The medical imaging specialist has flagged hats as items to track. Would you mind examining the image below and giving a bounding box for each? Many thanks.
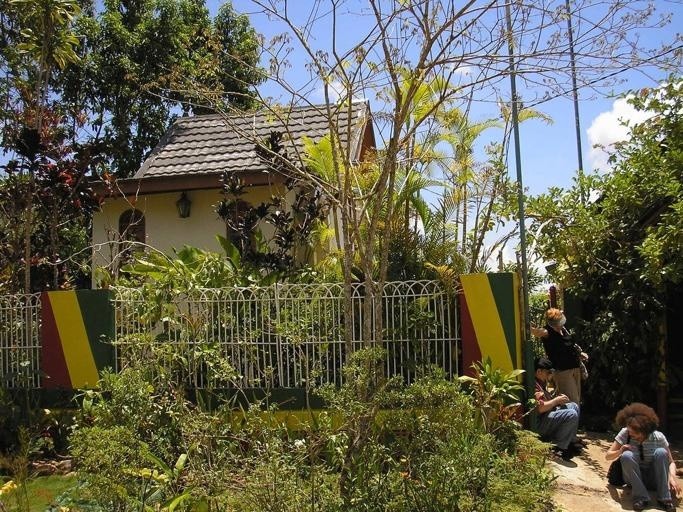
[534,358,556,371]
[548,312,566,326]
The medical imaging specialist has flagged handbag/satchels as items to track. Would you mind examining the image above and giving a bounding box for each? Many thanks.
[606,455,626,486]
[579,360,588,380]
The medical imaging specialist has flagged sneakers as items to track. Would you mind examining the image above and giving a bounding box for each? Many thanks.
[657,500,676,512]
[632,501,649,511]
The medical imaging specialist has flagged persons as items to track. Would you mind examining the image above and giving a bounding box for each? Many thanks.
[604,403,683,512]
[534,357,581,461]
[531,306,589,434]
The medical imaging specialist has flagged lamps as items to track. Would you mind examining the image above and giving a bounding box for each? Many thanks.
[175,192,191,219]
